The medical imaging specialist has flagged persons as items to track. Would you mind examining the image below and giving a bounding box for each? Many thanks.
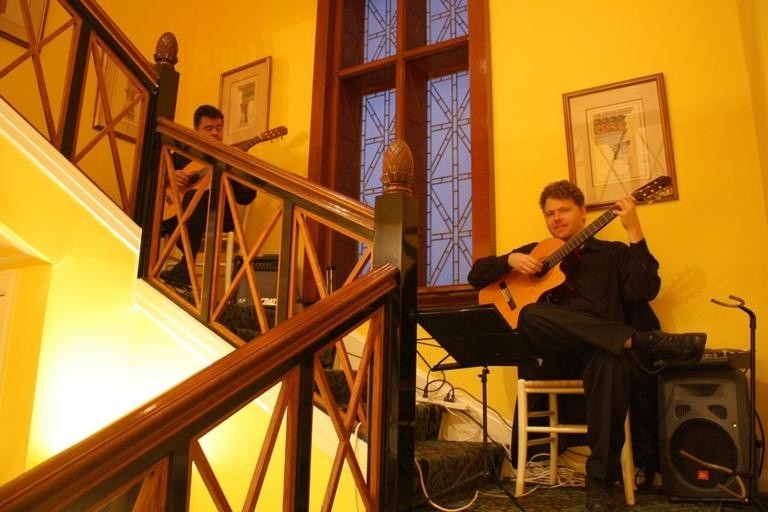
[467,180,707,512]
[160,105,256,292]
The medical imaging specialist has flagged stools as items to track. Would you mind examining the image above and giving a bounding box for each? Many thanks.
[515,379,636,505]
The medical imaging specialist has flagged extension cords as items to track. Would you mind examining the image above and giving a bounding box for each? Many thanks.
[415,396,469,411]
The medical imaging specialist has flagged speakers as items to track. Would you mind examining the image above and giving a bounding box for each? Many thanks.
[656,369,759,504]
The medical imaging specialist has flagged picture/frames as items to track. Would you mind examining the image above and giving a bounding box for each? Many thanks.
[0,0,50,52]
[218,54,272,146]
[92,51,142,143]
[562,71,681,211]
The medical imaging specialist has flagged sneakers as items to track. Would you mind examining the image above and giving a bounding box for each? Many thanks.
[586,489,619,512]
[635,329,706,366]
[159,258,190,284]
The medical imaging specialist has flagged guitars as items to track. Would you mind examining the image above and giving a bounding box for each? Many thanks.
[480,176,672,331]
[163,126,287,222]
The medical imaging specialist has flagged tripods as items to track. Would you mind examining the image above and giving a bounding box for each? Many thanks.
[412,369,526,512]
